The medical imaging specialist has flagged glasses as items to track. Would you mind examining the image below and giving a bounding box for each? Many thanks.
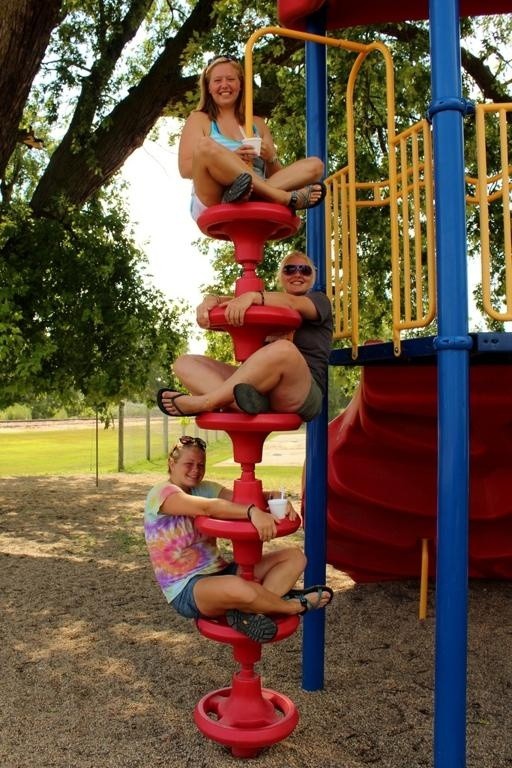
[282,264,313,276]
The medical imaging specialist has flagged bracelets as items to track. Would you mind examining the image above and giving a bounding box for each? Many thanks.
[269,492,272,499]
[247,504,255,521]
[259,291,264,306]
[212,295,221,304]
[268,154,278,164]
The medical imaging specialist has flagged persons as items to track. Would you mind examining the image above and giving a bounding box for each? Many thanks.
[158,252,333,422]
[178,57,326,223]
[144,436,334,644]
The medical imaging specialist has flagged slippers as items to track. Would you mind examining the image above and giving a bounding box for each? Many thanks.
[281,585,333,615]
[224,608,276,644]
[221,173,253,202]
[232,382,271,414]
[157,388,202,417]
[289,182,326,211]
